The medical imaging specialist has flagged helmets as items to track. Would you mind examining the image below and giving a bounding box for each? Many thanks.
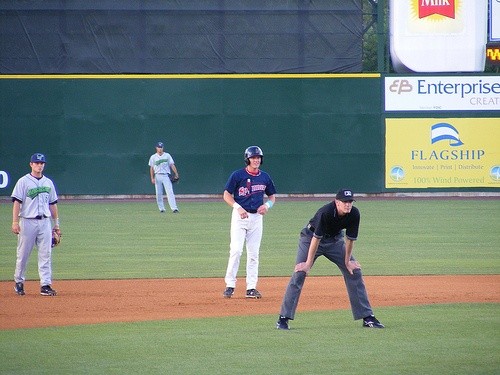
[244,146,264,165]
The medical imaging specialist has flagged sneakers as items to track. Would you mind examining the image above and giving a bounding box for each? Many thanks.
[276,316,290,330]
[223,287,234,297]
[363,315,384,328]
[14,282,25,296]
[40,285,57,295]
[246,289,261,297]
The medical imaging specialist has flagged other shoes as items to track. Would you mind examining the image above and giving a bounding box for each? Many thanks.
[161,210,165,213]
[174,210,179,213]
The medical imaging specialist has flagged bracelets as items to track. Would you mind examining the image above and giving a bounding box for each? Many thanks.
[12,222,19,225]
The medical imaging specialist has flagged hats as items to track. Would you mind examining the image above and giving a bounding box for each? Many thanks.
[31,153,46,163]
[336,189,355,201]
[156,142,164,147]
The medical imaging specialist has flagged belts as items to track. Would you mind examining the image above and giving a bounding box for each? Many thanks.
[307,223,315,232]
[36,216,46,219]
[247,210,257,213]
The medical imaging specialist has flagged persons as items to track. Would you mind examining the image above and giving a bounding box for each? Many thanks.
[277,188,385,331]
[222,146,277,299]
[9,153,62,296]
[148,142,180,213]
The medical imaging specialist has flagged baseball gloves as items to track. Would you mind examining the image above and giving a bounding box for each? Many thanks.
[52,229,63,248]
[169,171,182,184]
